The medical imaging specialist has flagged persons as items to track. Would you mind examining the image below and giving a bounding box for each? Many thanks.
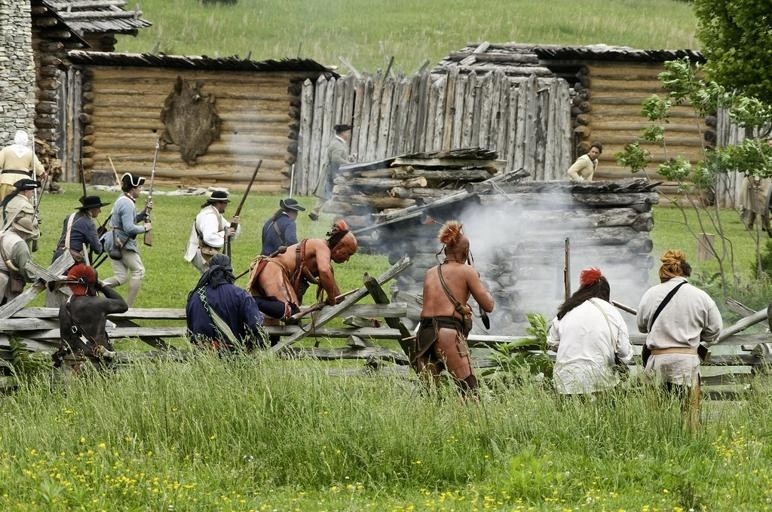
[308,125,354,221]
[546,267,634,405]
[261,198,305,257]
[44,196,111,308]
[186,253,268,361]
[566,143,602,180]
[59,263,128,376]
[637,250,723,428]
[100,172,153,308]
[0,179,41,256]
[0,130,46,202]
[0,216,38,307]
[415,218,495,404]
[184,191,241,275]
[246,220,358,348]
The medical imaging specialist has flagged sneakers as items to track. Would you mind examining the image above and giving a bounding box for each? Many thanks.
[309,213,318,220]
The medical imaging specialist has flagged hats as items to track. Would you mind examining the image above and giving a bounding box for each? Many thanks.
[121,172,146,186]
[210,191,230,203]
[12,217,34,235]
[74,196,110,209]
[14,180,41,191]
[334,125,353,132]
[280,199,307,213]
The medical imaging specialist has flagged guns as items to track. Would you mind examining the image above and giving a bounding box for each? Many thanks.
[610,300,711,362]
[460,227,489,329]
[89,214,112,264]
[24,136,39,216]
[144,141,160,246]
[285,288,359,324]
[223,160,262,265]
